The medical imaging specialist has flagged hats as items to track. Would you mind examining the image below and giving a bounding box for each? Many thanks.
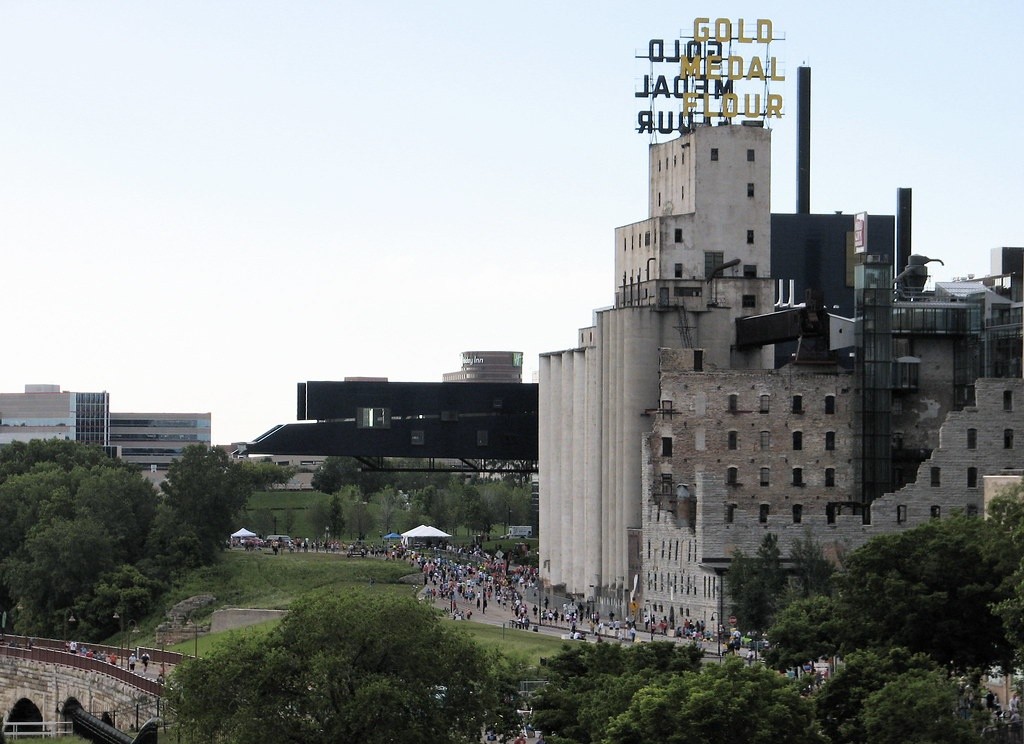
[132,653,134,656]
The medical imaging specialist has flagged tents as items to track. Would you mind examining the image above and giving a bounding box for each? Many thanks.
[384,533,401,542]
[402,525,452,540]
[231,527,257,548]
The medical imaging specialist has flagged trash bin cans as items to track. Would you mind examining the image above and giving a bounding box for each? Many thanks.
[532,625,538,632]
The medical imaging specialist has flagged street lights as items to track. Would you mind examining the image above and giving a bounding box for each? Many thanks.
[643,603,653,641]
[586,596,596,626]
[64,609,76,652]
[711,611,722,657]
[126,619,140,671]
[113,606,124,667]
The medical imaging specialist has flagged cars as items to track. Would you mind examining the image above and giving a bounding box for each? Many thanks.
[267,534,292,547]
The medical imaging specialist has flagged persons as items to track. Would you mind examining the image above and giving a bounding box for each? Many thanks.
[746,648,753,667]
[472,530,510,541]
[585,605,604,632]
[226,537,357,558]
[561,601,584,629]
[625,614,636,642]
[544,596,549,609]
[65,640,150,674]
[608,610,615,630]
[362,541,539,620]
[614,620,622,631]
[957,685,1024,744]
[644,615,648,630]
[723,629,741,657]
[762,635,770,649]
[651,614,656,636]
[676,618,712,645]
[568,629,587,641]
[658,616,668,637]
[785,658,829,693]
[533,605,559,626]
[494,582,530,631]
